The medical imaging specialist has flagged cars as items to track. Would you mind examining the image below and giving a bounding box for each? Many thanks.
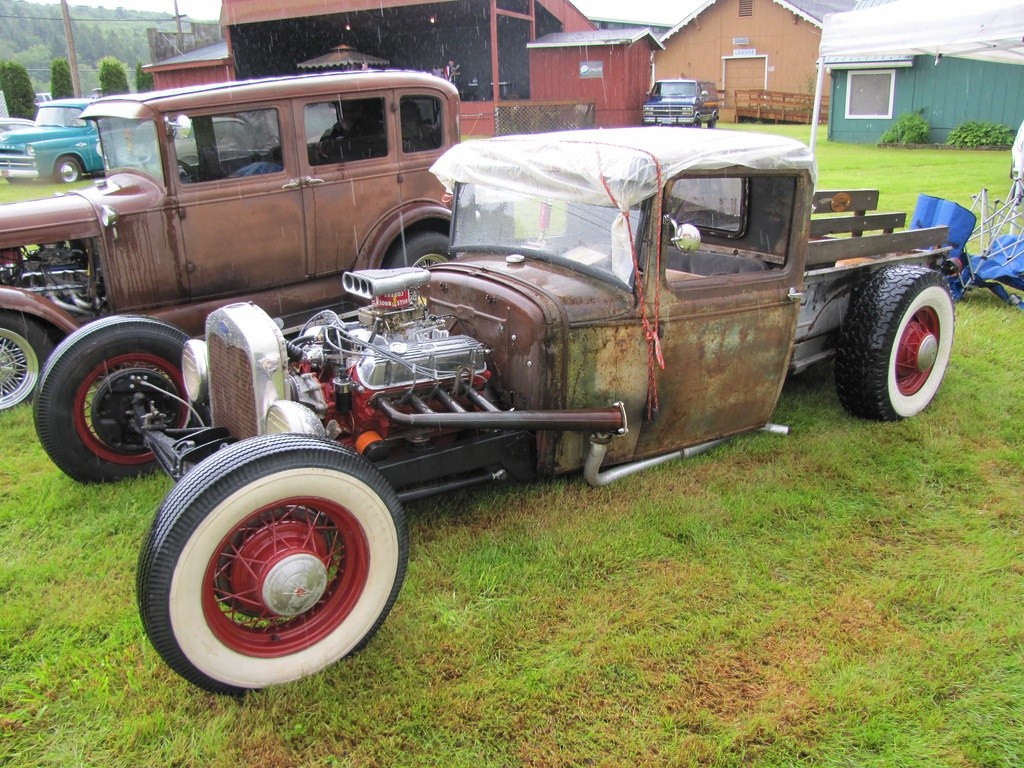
[31,91,55,105]
[0,97,172,185]
[0,66,470,413]
[0,117,35,146]
[32,128,954,693]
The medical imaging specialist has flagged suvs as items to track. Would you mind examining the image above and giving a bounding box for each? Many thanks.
[638,79,722,134]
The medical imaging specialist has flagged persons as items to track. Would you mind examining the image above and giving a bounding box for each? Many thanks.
[226,143,285,180]
